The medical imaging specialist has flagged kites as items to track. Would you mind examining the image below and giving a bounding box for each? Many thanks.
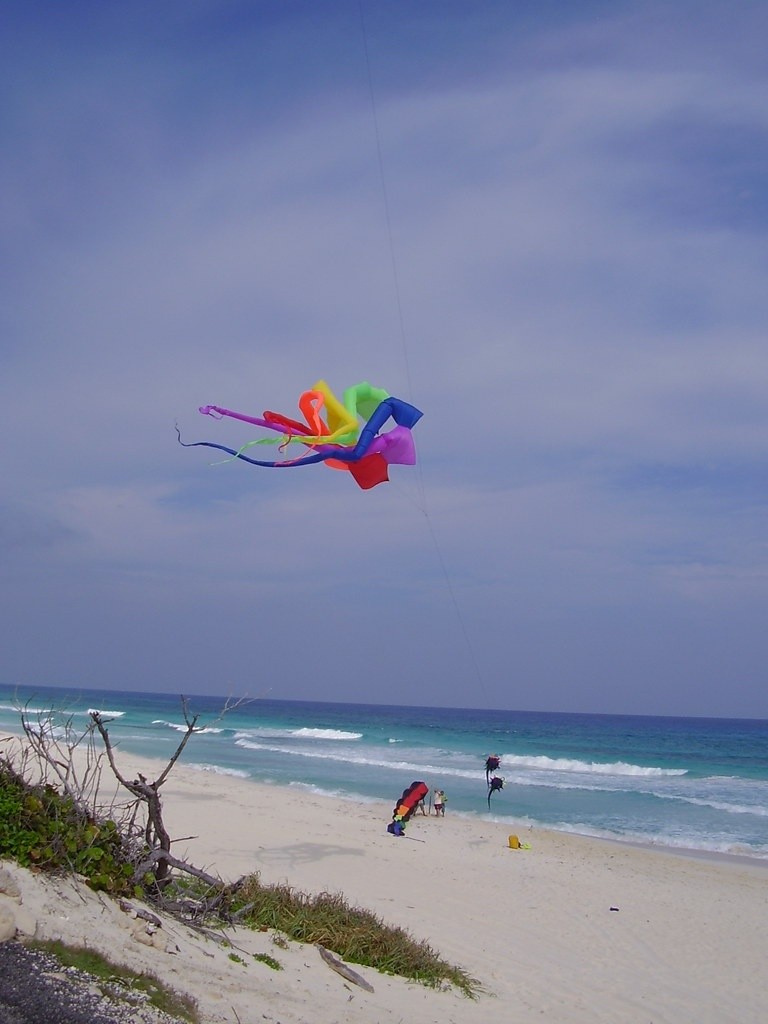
[175,380,424,490]
[487,775,504,810]
[483,756,500,788]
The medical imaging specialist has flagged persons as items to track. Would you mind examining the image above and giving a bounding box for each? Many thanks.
[392,819,405,836]
[413,798,427,816]
[434,788,447,817]
